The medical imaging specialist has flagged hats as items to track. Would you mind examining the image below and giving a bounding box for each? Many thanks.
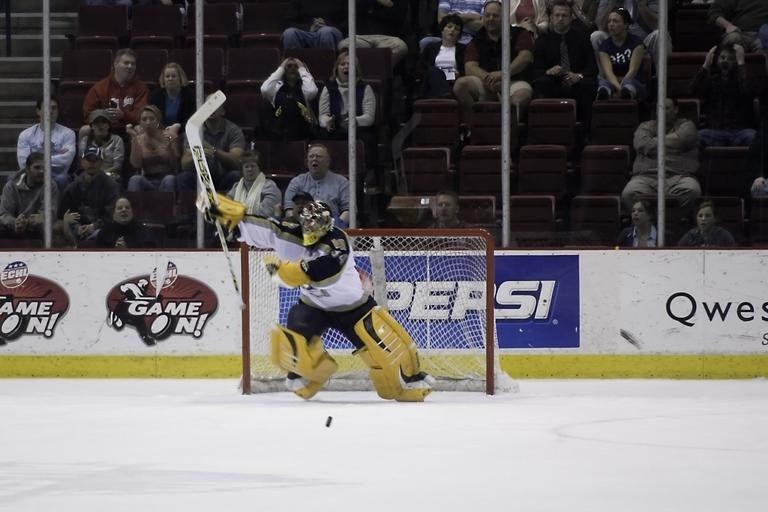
[292,191,313,203]
[89,110,110,125]
[82,146,103,161]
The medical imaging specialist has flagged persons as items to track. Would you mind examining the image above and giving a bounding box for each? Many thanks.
[1,0,767,262]
[205,200,436,391]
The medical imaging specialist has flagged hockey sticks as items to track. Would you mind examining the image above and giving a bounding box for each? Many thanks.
[186,90,246,311]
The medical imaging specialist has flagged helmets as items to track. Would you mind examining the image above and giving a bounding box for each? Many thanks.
[301,199,335,246]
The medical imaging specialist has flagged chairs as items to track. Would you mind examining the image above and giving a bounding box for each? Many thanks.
[54,0,767,247]
